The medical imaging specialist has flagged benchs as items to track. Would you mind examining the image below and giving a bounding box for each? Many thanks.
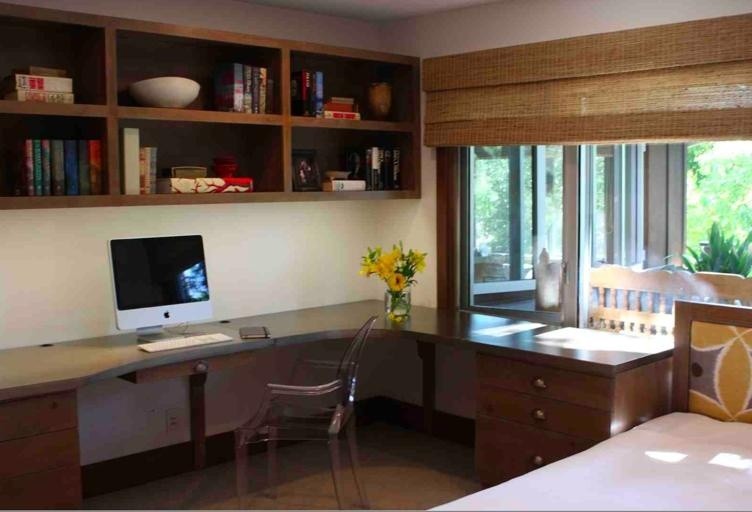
[534,249,752,340]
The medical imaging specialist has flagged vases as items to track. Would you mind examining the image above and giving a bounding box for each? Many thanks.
[384,289,412,323]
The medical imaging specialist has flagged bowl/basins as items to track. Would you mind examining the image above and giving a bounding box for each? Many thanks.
[130,75,200,108]
[214,150,241,178]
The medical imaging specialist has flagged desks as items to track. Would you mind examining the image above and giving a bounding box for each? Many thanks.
[0,298,676,511]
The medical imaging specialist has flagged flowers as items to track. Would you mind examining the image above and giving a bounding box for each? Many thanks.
[361,240,428,313]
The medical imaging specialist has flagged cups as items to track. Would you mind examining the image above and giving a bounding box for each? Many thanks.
[369,83,394,123]
[535,261,562,312]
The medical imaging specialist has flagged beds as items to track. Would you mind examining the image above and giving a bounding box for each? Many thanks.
[428,298,751,512]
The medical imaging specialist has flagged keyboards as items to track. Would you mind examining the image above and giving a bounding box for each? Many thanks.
[137,333,233,352]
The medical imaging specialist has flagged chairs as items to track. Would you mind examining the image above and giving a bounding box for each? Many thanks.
[233,314,378,512]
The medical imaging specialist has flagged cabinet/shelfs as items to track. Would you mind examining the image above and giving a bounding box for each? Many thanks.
[0,2,421,212]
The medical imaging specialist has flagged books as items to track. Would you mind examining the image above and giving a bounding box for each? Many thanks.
[4,91,76,104]
[2,127,254,197]
[239,326,271,339]
[322,146,400,191]
[12,65,76,78]
[1,75,75,92]
[214,62,361,120]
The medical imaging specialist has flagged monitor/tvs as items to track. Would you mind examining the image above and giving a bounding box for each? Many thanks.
[108,234,210,341]
